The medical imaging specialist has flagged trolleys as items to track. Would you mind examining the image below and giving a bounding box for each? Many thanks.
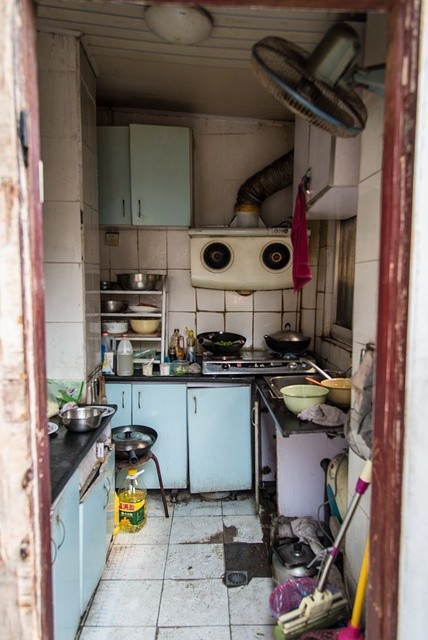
[249,23,388,137]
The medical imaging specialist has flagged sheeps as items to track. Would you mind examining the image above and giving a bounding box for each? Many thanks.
[299,358,332,379]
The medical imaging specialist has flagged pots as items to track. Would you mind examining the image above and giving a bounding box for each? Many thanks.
[197,331,246,355]
[264,323,311,353]
[111,424,159,465]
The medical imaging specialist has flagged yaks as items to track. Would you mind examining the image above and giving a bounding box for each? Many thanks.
[299,520,371,640]
[273,459,372,640]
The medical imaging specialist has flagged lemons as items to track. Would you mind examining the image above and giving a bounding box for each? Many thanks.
[271,542,319,587]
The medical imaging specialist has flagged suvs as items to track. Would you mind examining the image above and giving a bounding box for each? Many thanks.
[101,331,116,376]
[117,468,147,533]
[170,329,184,360]
[116,333,134,377]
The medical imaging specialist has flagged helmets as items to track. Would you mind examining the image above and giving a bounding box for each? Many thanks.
[128,305,158,313]
[83,406,115,417]
[47,422,58,435]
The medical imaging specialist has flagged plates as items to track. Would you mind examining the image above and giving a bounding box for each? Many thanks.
[186,386,253,495]
[95,124,192,228]
[79,440,116,615]
[292,114,361,221]
[98,284,167,364]
[50,467,81,640]
[100,383,187,491]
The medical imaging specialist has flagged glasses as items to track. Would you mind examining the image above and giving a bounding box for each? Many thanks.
[143,4,213,45]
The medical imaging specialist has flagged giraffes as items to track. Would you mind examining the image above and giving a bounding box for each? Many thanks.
[202,350,317,375]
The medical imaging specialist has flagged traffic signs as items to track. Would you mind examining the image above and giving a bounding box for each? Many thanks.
[105,233,119,246]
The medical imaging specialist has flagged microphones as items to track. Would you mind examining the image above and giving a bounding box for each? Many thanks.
[116,450,169,518]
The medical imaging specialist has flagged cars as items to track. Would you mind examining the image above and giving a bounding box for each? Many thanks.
[161,363,170,375]
[143,360,152,376]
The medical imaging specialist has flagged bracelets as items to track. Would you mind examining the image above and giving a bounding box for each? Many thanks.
[99,280,116,290]
[116,273,166,290]
[101,321,128,334]
[280,385,329,415]
[130,320,160,334]
[100,300,129,313]
[59,407,105,432]
[321,378,351,404]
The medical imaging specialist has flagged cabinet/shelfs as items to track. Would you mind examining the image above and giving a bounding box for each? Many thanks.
[119,468,148,533]
[186,328,197,362]
[117,334,134,376]
[170,329,184,361]
[101,331,116,375]
[113,491,118,535]
